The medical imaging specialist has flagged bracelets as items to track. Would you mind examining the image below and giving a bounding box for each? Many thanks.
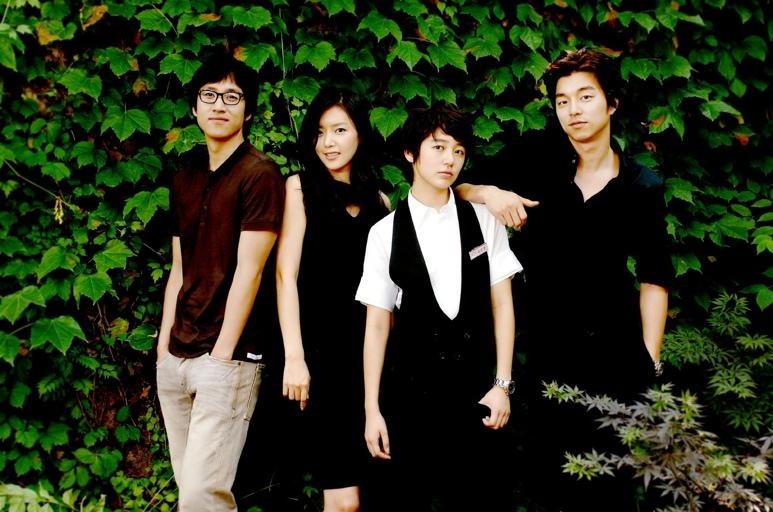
[653,357,665,377]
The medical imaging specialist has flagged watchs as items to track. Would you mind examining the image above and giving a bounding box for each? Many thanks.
[491,377,517,395]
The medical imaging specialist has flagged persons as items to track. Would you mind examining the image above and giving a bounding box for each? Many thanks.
[452,48,677,512]
[152,55,288,511]
[273,82,393,512]
[353,105,523,512]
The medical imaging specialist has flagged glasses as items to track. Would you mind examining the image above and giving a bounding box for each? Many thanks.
[194,89,246,105]
